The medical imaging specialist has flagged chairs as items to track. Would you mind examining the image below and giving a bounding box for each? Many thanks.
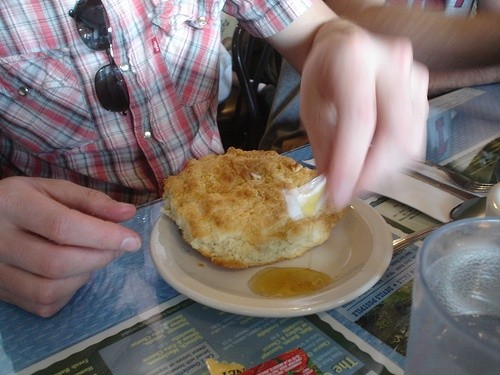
[232,22,282,151]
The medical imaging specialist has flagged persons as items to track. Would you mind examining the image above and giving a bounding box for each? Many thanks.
[259,1,500,156]
[0,0,431,318]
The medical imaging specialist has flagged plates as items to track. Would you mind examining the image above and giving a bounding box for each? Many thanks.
[150,198,393,317]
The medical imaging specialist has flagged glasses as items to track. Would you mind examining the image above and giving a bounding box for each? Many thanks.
[68,0,130,116]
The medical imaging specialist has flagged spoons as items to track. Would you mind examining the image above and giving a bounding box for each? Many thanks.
[393,197,486,254]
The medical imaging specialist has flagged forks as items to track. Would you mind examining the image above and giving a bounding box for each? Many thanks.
[415,159,493,190]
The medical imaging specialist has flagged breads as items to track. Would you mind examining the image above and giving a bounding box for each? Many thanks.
[160,147,353,267]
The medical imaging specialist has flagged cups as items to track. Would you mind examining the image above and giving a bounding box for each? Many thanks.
[405,216,500,375]
